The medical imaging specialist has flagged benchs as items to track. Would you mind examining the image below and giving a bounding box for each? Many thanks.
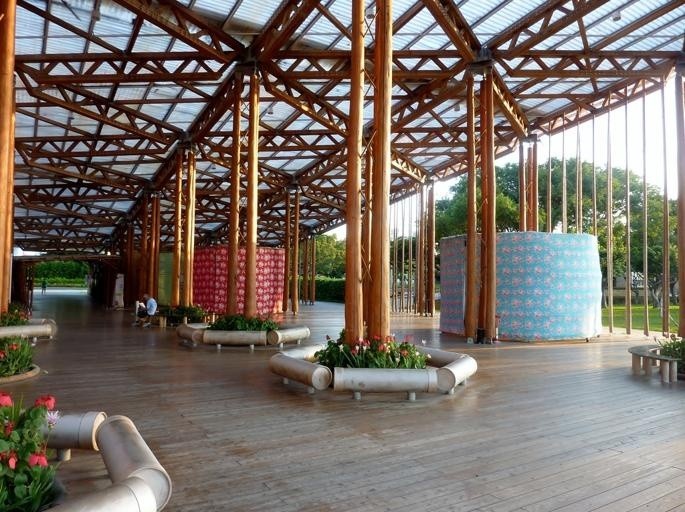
[627,344,683,383]
[153,312,168,328]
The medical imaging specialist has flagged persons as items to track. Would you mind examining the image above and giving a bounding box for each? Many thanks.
[41,278,48,296]
[131,291,158,328]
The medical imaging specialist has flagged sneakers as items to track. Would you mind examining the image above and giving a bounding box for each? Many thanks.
[144,323,151,327]
[132,322,138,326]
